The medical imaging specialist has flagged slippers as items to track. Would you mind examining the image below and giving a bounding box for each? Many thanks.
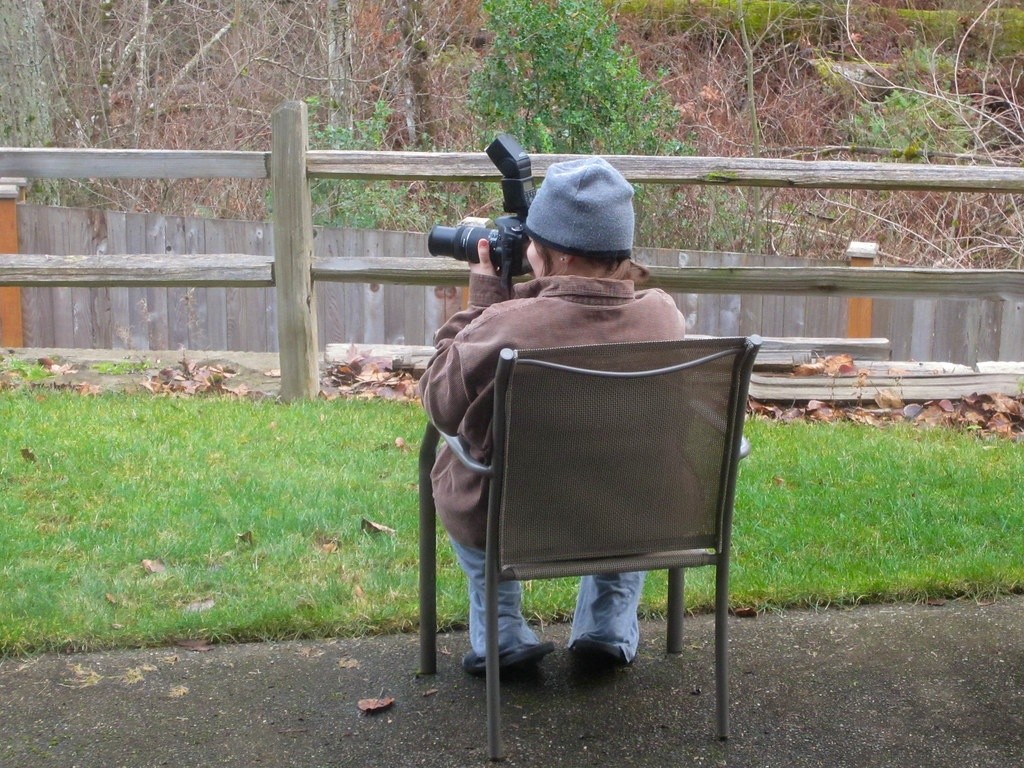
[572,639,626,665]
[461,640,554,673]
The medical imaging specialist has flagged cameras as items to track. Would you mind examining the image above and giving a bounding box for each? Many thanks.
[428,135,539,277]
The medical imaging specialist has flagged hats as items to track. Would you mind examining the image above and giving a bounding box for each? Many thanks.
[526,158,634,257]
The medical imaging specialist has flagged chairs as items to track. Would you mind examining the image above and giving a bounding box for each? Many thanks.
[418,333,765,761]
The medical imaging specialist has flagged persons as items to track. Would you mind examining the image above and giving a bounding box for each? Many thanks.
[416,156,686,674]
